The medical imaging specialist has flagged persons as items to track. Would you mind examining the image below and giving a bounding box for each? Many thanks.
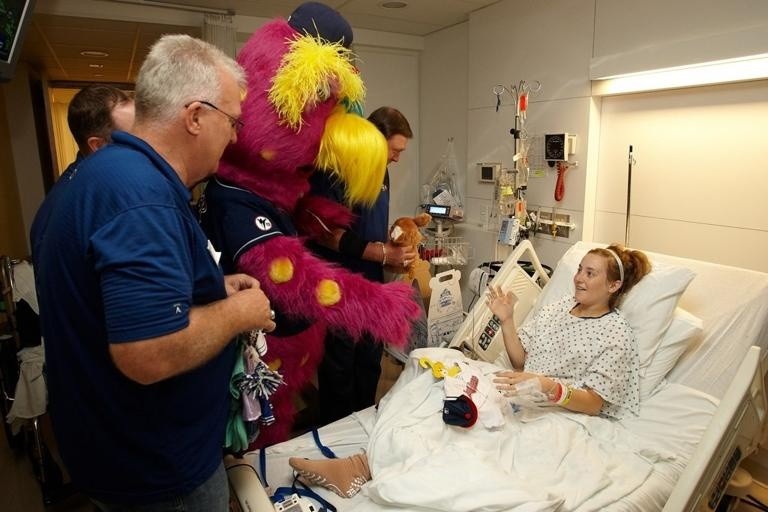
[27,32,279,512]
[312,107,416,423]
[289,243,651,498]
[51,84,136,186]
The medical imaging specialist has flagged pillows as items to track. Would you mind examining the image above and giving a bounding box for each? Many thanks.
[534,240,704,400]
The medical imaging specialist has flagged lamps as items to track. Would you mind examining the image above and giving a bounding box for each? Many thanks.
[591,53,768,96]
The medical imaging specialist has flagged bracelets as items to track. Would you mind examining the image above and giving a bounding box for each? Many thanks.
[555,382,573,407]
[375,241,387,265]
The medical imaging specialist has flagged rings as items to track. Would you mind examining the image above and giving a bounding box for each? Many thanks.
[403,259,408,268]
[269,309,276,320]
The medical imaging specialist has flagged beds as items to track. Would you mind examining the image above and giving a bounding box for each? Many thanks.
[222,241,767,511]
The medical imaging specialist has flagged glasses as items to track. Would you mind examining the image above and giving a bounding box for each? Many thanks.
[184,101,244,133]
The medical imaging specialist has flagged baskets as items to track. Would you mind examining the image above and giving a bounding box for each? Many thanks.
[418,236,471,265]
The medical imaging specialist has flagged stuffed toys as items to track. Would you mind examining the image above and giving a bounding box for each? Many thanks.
[384,213,432,274]
[195,1,423,454]
